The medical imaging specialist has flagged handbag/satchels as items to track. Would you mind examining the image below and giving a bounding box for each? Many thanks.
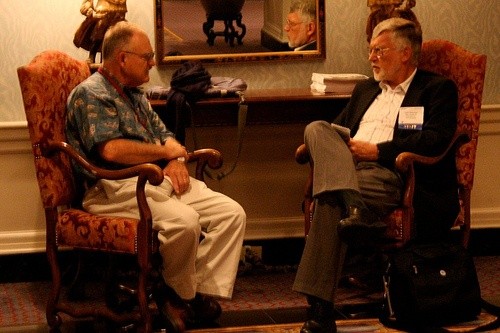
[378,230,500,333]
[144,76,248,181]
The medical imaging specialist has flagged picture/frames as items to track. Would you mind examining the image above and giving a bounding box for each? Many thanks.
[153,0,327,69]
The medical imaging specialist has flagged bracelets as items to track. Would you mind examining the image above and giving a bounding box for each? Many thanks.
[177,157,185,162]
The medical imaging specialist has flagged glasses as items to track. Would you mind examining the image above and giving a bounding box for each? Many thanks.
[119,50,155,60]
[368,45,395,57]
[283,19,306,29]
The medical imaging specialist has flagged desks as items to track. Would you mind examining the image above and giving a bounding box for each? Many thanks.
[147,87,352,145]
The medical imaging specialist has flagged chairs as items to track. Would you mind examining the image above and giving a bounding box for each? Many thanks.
[294,38,487,287]
[17,52,224,333]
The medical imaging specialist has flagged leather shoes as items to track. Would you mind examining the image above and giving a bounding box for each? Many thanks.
[297,304,337,332]
[339,204,387,233]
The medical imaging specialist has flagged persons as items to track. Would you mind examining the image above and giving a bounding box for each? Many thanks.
[64,21,247,331]
[292,17,460,333]
[284,1,317,50]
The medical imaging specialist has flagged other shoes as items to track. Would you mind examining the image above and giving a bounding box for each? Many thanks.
[190,293,221,326]
[156,286,190,333]
